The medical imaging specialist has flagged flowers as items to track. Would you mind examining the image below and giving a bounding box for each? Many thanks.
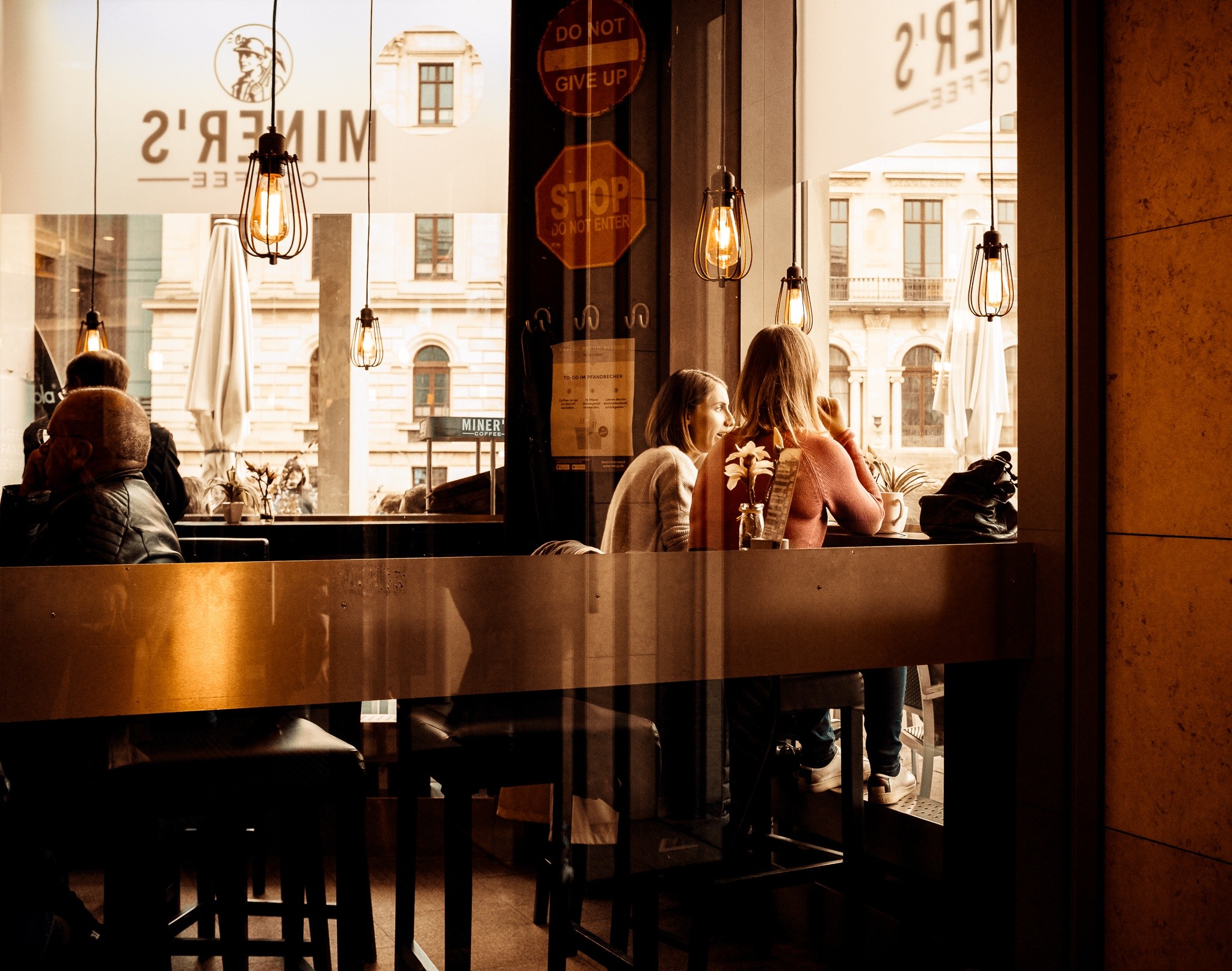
[246,463,281,499]
[725,431,784,507]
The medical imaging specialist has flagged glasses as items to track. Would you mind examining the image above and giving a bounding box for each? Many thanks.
[37,429,91,445]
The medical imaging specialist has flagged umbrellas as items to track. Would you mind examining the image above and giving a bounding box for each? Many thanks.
[183,218,256,507]
[932,219,1018,469]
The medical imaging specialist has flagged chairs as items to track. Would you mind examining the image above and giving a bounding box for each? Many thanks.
[90,534,851,971]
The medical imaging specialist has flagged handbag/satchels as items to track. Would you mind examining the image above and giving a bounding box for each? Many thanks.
[919,450,1019,542]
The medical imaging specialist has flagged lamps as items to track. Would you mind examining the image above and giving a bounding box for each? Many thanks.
[350,0,383,371]
[774,0,814,337]
[692,0,753,289]
[74,0,109,357]
[237,0,309,266]
[967,0,1013,322]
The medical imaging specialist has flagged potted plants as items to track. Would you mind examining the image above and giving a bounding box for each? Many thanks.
[197,469,261,524]
[863,453,935,534]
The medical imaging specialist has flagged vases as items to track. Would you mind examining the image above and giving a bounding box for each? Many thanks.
[257,497,275,525]
[738,503,766,550]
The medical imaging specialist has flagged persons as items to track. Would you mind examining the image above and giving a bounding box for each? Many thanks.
[269,458,317,515]
[594,327,909,811]
[23,348,189,524]
[1,385,185,971]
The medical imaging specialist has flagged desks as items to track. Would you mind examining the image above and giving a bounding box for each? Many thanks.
[174,511,500,560]
[821,520,927,546]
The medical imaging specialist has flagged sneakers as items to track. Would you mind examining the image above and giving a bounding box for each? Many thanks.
[868,760,916,804]
[798,745,871,793]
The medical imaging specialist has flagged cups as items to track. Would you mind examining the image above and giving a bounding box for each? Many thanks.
[881,493,905,533]
